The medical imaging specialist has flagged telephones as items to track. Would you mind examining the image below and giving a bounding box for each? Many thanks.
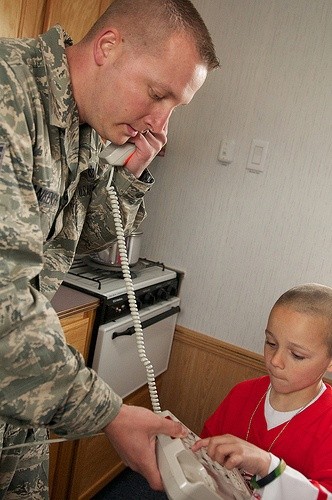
[98,142,137,166]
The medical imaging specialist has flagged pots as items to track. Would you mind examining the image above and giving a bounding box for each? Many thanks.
[89,230,143,267]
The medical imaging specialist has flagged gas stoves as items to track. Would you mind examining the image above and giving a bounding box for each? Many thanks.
[61,258,185,300]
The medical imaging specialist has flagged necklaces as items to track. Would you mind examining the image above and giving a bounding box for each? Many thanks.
[245,384,322,453]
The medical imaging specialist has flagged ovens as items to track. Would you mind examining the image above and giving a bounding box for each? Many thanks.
[91,297,185,400]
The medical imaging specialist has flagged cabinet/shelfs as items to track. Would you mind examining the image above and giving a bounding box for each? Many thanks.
[48,286,101,500]
[67,377,165,500]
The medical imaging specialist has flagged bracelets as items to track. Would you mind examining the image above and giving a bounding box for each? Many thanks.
[250,458,287,489]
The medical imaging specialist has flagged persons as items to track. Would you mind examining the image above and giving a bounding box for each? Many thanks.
[189,283,332,500]
[0,0,220,500]
